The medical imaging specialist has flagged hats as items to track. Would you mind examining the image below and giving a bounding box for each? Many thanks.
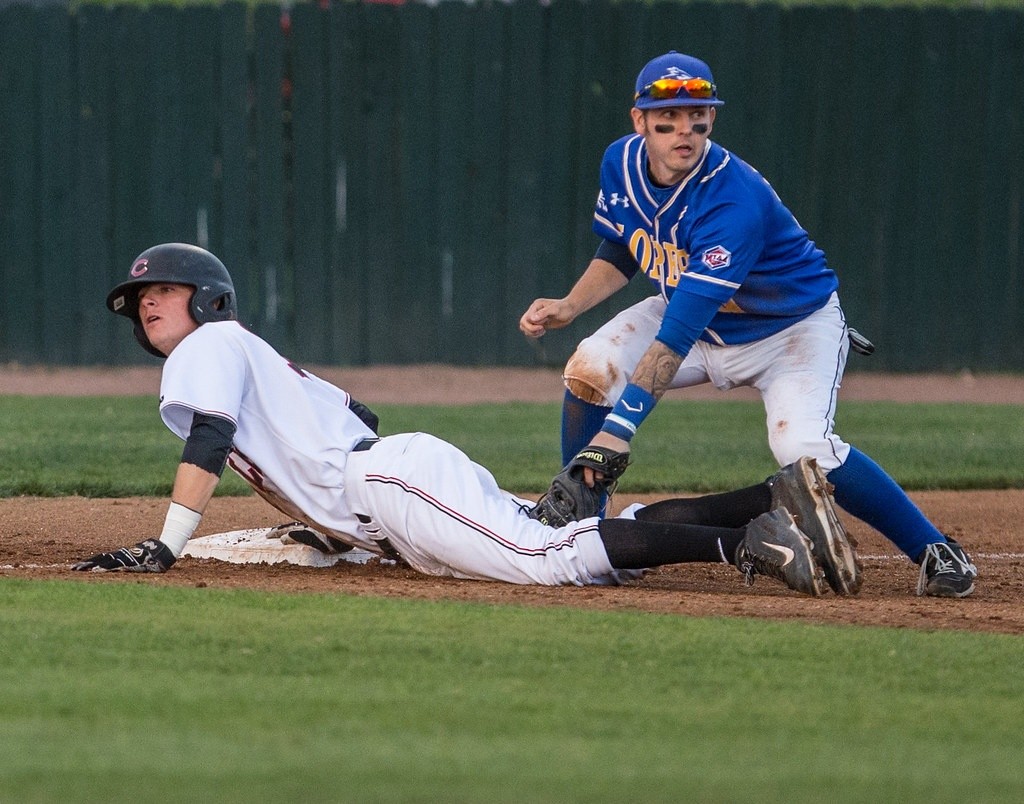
[635,50,725,109]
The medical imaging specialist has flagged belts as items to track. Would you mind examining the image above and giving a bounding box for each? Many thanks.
[352,438,410,569]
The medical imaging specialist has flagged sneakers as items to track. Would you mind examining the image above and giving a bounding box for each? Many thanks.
[916,535,978,598]
[764,456,864,598]
[735,505,829,599]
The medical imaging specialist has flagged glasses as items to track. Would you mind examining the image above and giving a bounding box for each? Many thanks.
[633,77,718,105]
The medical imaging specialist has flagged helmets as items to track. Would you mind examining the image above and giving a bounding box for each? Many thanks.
[105,242,238,358]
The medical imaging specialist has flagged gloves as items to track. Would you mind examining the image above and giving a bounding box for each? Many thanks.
[70,539,177,573]
[848,327,874,356]
[265,521,354,555]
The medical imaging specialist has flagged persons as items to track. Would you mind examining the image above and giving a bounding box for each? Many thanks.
[517,50,977,600]
[71,243,864,599]
